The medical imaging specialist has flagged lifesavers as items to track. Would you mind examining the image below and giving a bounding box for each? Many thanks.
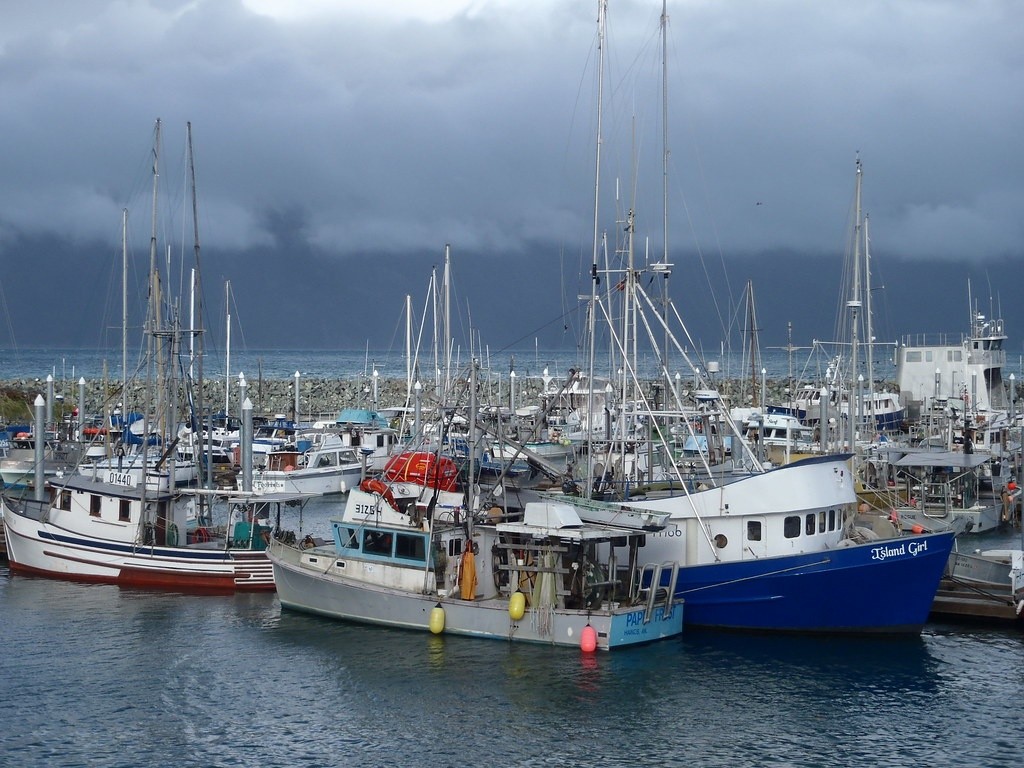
[359,477,396,506]
[192,528,207,544]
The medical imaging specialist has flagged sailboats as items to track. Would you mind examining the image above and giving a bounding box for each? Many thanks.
[0,0,1024,653]
